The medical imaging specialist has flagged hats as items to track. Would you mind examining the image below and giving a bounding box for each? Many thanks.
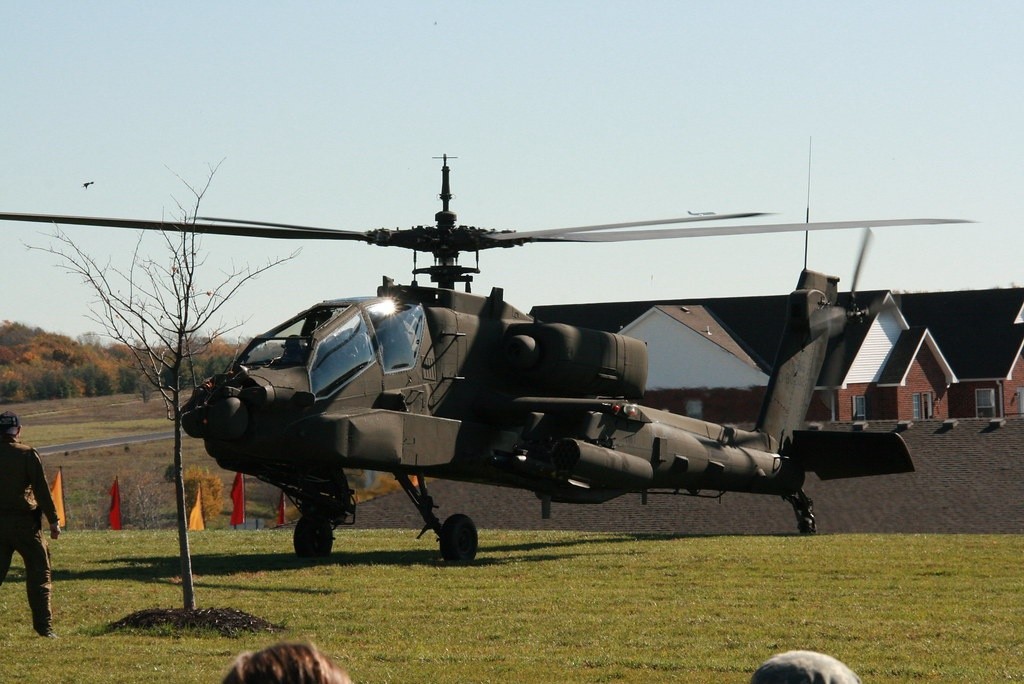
[749,650,862,684]
[0,411,20,434]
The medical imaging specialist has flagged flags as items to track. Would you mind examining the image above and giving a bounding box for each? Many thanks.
[49,466,286,530]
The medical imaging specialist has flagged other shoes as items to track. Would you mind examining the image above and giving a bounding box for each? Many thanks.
[33,627,58,638]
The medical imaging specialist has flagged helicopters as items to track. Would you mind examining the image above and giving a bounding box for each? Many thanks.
[0,154,977,568]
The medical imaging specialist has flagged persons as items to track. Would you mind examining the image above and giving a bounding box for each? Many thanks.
[221,643,354,684]
[749,650,862,684]
[0,411,61,640]
